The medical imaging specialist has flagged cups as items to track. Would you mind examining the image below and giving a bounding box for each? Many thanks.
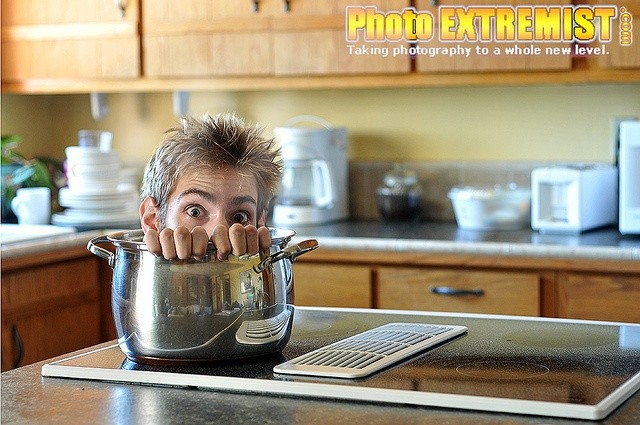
[9,187,51,225]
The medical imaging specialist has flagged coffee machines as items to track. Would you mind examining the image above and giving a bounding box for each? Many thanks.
[270,117,350,227]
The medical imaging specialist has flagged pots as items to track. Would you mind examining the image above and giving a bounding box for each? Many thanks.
[86,226,319,368]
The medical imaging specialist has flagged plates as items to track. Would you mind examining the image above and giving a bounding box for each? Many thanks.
[50,146,141,227]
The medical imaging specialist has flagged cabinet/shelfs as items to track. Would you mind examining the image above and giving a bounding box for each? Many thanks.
[414,1,579,87]
[141,1,413,93]
[375,263,553,318]
[284,261,375,310]
[1,256,104,371]
[1,1,141,95]
[552,269,640,324]
[578,1,639,83]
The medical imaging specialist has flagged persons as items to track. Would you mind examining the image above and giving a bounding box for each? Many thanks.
[138,113,285,260]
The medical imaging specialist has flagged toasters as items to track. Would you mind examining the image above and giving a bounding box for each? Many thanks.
[531,162,618,234]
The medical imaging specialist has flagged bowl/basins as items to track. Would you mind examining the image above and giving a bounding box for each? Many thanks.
[448,184,530,230]
